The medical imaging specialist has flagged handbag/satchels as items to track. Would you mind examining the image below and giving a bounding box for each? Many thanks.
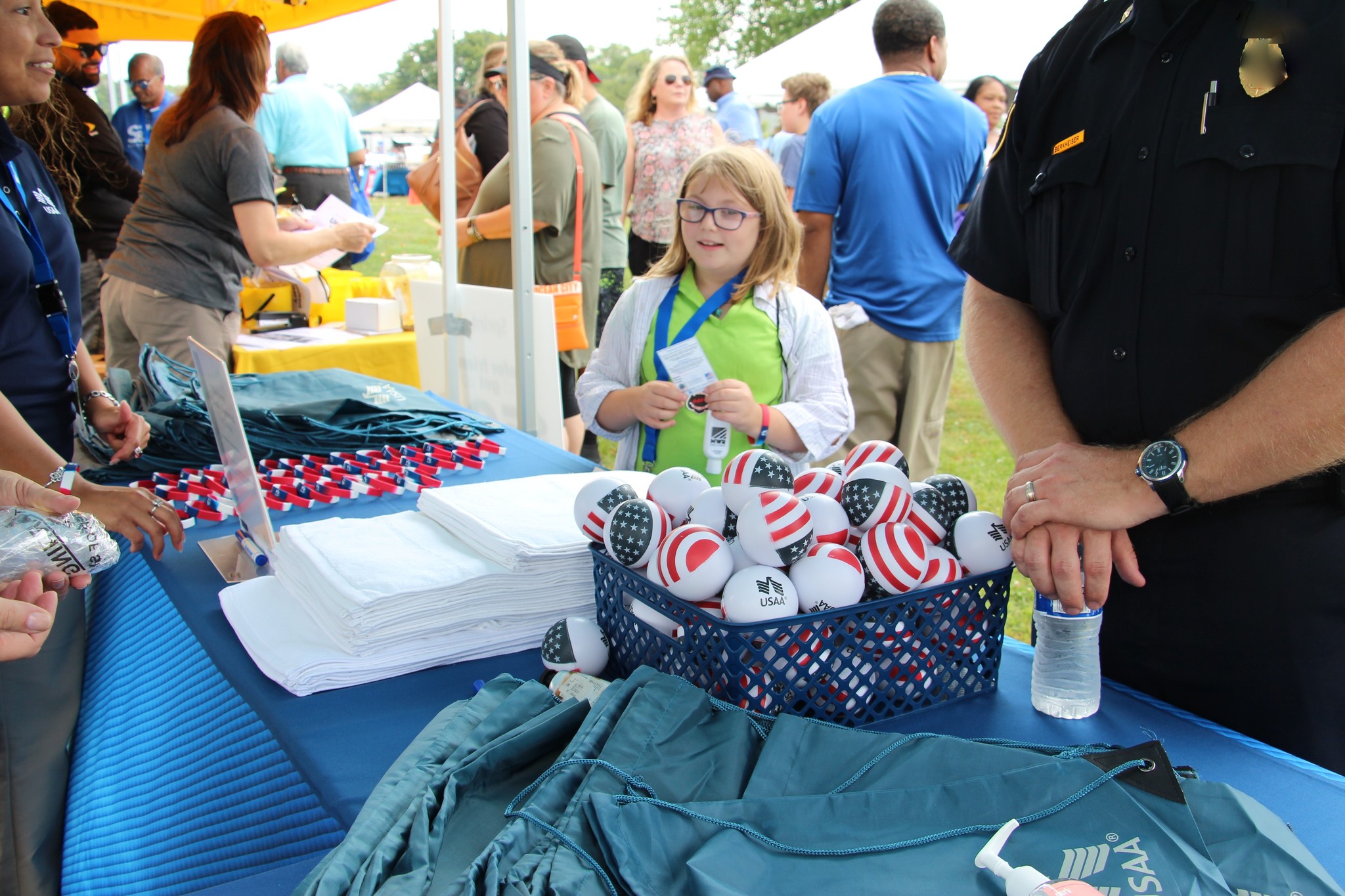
[81,342,506,484]
[406,99,493,225]
[298,662,1345,894]
[535,280,590,351]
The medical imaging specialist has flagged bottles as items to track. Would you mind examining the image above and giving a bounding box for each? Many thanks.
[1031,540,1103,720]
[379,253,444,331]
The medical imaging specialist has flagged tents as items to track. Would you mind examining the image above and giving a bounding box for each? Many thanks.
[353,81,442,169]
[732,0,1086,122]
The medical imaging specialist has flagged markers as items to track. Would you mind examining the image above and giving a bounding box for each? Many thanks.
[236,529,267,566]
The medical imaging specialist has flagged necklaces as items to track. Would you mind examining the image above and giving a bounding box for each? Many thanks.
[714,301,731,317]
[881,70,927,76]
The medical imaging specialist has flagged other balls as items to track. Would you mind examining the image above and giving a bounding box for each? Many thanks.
[540,616,610,679]
[572,438,1024,720]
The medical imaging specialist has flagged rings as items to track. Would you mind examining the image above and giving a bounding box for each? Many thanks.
[1024,481,1036,503]
[134,447,144,459]
[151,497,163,515]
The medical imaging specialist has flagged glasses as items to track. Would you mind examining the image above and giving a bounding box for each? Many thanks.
[126,77,157,90]
[664,75,691,85]
[251,14,268,39]
[59,40,111,58]
[675,198,758,231]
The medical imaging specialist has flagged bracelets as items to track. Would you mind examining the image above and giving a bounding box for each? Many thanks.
[128,435,507,536]
[42,466,65,488]
[748,404,770,447]
[81,389,119,427]
[58,460,80,494]
[465,216,484,243]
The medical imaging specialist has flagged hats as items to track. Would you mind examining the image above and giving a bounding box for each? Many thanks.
[703,66,736,85]
[484,53,567,84]
[547,34,601,82]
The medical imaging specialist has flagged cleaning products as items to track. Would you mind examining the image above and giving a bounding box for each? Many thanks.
[974,817,1104,896]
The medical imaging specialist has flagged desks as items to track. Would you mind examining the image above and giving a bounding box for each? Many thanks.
[232,322,422,392]
[63,370,1344,895]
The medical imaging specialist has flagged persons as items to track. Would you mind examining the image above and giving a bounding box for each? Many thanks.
[1,470,92,661]
[947,1,1345,774]
[100,10,376,381]
[575,143,854,482]
[624,43,727,278]
[762,76,831,225]
[702,65,762,148]
[110,53,179,177]
[0,0,186,896]
[964,74,1008,175]
[795,0,987,483]
[430,35,630,466]
[254,44,367,271]
[6,0,143,356]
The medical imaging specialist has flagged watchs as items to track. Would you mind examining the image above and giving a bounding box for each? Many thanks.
[1134,429,1200,517]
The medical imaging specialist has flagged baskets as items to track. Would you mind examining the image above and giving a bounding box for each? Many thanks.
[586,539,1018,728]
